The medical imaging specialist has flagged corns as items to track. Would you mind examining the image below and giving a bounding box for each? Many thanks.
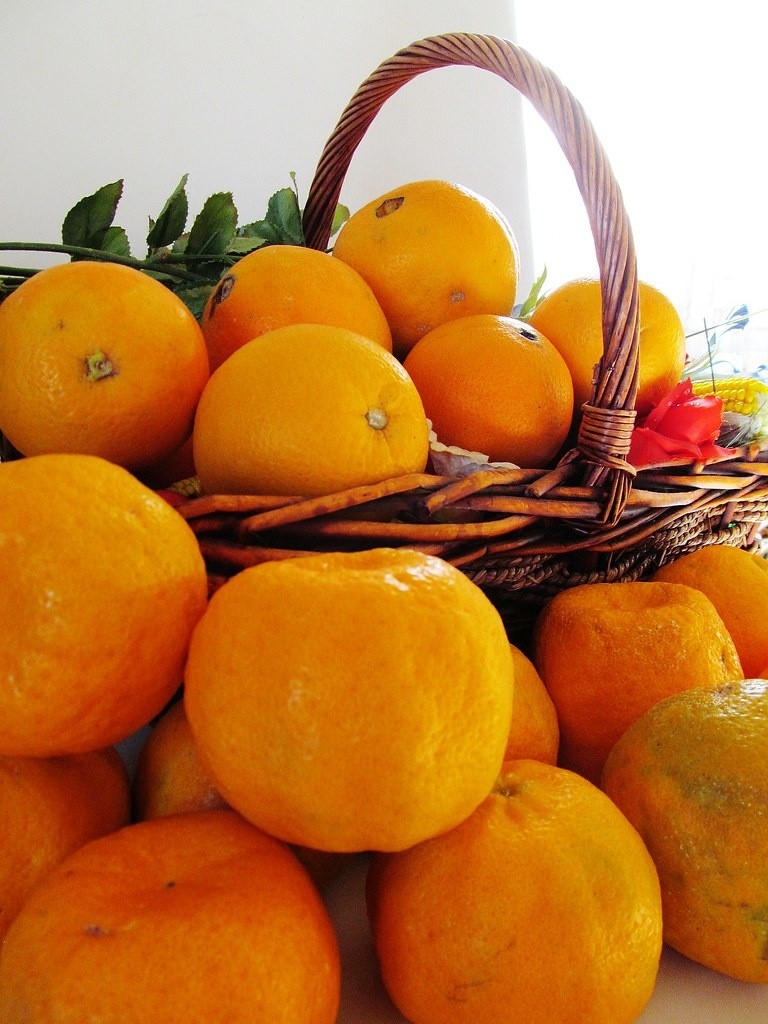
[692,379,768,415]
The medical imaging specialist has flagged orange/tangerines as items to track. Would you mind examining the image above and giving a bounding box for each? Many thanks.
[0,455,768,1024]
[0,179,686,498]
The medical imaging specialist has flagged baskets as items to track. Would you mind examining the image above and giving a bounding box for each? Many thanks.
[154,34,768,645]
